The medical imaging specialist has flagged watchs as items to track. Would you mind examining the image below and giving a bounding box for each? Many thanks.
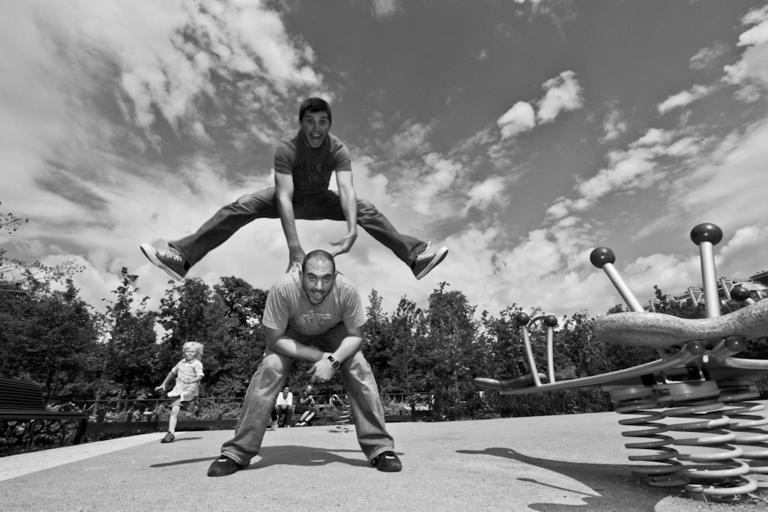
[328,355,341,370]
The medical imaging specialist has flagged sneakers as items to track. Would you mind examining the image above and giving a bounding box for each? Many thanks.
[413,245,448,280]
[370,450,402,472]
[207,455,249,477]
[160,431,174,443]
[140,243,189,281]
[271,421,305,431]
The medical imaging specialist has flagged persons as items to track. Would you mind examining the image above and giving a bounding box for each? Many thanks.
[205,249,403,477]
[274,384,295,428]
[139,97,450,282]
[153,340,206,444]
[296,382,318,428]
